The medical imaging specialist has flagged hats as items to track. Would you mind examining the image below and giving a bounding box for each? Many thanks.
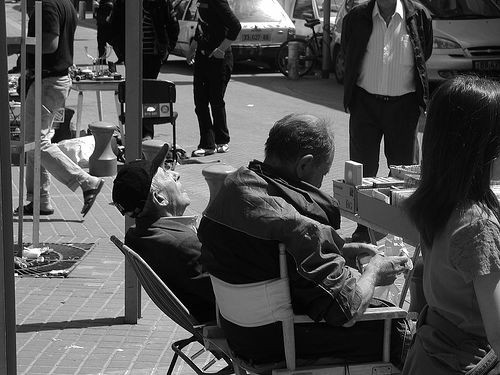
[112,143,170,218]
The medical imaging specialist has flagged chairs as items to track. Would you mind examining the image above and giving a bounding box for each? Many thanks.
[111,235,244,375]
[117,79,178,163]
[197,214,406,375]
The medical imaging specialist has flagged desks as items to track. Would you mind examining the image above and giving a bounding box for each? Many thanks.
[67,78,126,139]
[340,210,421,310]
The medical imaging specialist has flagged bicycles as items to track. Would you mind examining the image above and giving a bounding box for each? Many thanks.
[277,19,333,80]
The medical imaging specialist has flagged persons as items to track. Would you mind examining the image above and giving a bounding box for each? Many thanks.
[340,0,433,242]
[8,0,105,218]
[93,0,181,141]
[401,76,500,375]
[196,112,415,371]
[111,144,214,326]
[187,0,243,157]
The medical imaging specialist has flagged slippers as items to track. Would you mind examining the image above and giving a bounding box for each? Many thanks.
[81,179,104,217]
[13,204,54,215]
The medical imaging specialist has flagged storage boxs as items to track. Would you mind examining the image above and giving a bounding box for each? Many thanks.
[333,180,424,244]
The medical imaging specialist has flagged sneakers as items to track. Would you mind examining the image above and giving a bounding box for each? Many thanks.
[192,144,216,158]
[217,143,229,153]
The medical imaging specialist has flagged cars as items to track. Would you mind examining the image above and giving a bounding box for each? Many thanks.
[285,1,345,45]
[170,0,296,73]
[330,0,500,99]
